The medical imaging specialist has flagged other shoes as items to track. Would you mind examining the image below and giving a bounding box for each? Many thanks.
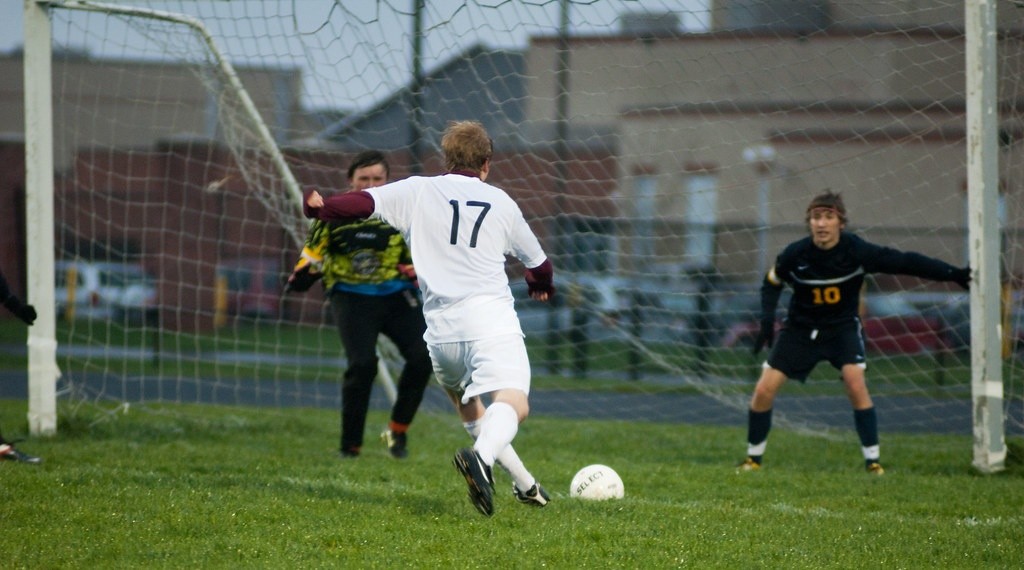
[866,458,884,476]
[736,457,761,471]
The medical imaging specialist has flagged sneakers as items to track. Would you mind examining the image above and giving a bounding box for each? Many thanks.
[0,441,41,463]
[512,480,551,506]
[452,447,496,518]
[381,428,408,459]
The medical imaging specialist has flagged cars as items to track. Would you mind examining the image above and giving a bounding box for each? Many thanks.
[53,257,150,321]
[719,287,952,359]
[512,272,675,348]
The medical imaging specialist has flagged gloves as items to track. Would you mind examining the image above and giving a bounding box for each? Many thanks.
[752,273,786,356]
[896,246,972,293]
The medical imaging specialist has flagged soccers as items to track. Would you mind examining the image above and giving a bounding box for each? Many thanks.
[570,464,624,501]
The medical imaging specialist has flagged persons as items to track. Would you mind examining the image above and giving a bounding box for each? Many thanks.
[303,118,554,518]
[286,149,433,459]
[735,187,973,476]
